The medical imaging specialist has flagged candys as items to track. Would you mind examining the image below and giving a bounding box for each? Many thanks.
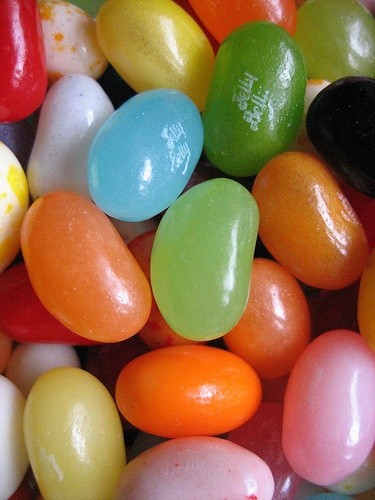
[0,0,375,500]
